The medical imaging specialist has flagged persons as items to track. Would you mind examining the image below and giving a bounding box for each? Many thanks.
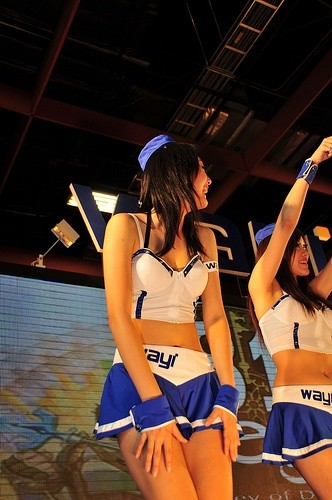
[103,134,241,500]
[248,135,332,500]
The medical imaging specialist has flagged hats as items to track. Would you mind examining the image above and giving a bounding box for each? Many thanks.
[255,223,275,244]
[138,135,176,171]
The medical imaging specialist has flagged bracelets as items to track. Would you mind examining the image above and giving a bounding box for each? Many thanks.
[128,393,175,433]
[213,384,240,422]
[296,158,318,185]
